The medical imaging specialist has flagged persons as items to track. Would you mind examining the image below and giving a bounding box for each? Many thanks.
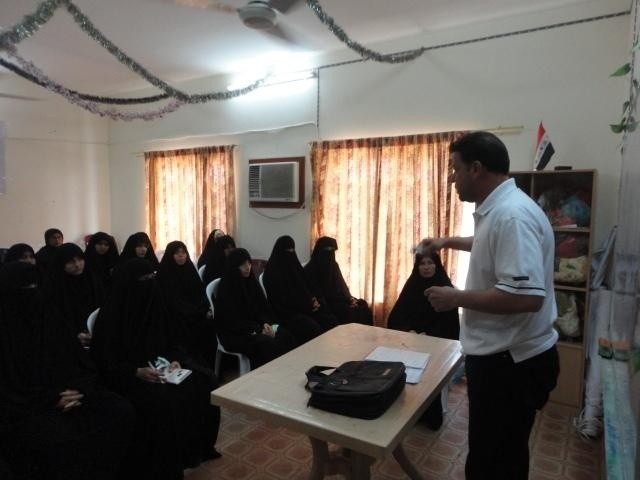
[410,128,560,480]
[1,223,223,480]
[196,222,377,371]
[387,249,461,432]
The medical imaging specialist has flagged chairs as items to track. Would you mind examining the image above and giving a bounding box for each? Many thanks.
[87,261,323,377]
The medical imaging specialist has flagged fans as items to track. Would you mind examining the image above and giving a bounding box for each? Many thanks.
[170,0,298,43]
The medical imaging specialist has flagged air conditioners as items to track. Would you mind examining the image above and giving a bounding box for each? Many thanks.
[249,161,300,203]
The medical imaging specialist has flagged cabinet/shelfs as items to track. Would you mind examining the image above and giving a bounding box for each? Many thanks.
[507,168,599,408]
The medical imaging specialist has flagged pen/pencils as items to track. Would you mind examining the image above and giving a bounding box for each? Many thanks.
[148,362,164,384]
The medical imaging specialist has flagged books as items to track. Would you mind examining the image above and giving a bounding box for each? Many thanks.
[364,346,431,385]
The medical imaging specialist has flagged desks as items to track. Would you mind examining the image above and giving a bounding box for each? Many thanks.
[210,319,466,479]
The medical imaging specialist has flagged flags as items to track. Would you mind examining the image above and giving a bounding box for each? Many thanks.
[531,121,555,172]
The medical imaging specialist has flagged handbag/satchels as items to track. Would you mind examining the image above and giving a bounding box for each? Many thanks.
[305,360,406,420]
